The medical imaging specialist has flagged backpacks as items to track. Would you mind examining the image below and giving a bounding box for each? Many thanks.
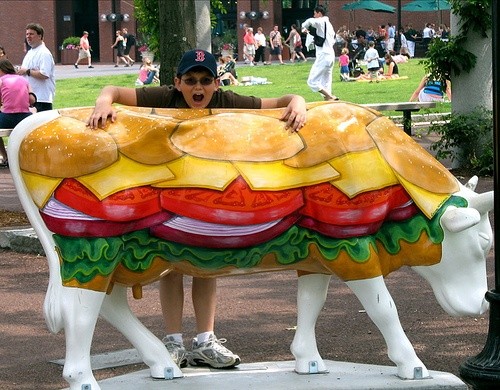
[126,34,136,46]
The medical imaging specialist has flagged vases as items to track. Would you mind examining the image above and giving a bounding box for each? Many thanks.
[220,49,235,59]
[61,48,89,65]
[139,51,156,64]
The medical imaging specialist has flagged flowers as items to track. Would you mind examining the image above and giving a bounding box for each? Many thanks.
[222,42,236,50]
[66,44,93,51]
[138,44,148,51]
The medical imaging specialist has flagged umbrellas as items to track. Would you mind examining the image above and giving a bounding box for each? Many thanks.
[340,0,397,30]
[400,0,454,28]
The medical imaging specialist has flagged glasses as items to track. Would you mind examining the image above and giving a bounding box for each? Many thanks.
[180,75,214,85]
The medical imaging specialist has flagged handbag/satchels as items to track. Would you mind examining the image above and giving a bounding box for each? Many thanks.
[314,33,325,47]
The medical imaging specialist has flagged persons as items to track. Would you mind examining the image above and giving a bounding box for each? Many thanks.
[110,27,135,66]
[74,30,94,69]
[409,65,451,103]
[242,23,268,66]
[267,26,286,64]
[85,48,307,370]
[138,57,161,86]
[0,23,55,169]
[215,55,237,86]
[300,4,339,101]
[283,24,307,63]
[335,22,450,84]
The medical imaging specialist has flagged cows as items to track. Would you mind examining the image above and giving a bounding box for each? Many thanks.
[6,100,494,389]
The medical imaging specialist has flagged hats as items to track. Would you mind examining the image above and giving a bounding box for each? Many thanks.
[177,50,218,81]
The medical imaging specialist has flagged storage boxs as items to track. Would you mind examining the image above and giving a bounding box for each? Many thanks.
[378,58,386,64]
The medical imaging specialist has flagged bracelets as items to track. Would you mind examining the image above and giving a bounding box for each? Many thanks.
[26,69,30,76]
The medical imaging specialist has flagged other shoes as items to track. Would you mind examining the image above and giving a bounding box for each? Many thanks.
[0,160,9,169]
[88,66,94,68]
[114,65,118,67]
[298,56,301,62]
[131,61,134,66]
[74,63,77,68]
[125,64,129,67]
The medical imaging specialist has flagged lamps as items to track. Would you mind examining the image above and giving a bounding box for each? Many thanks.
[240,10,270,20]
[100,12,130,24]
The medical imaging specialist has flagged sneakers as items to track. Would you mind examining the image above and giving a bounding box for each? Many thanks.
[162,337,187,368]
[188,332,242,369]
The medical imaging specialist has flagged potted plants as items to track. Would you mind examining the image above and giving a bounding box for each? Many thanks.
[264,34,290,62]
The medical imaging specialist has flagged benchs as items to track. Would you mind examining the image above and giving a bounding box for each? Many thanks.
[0,129,13,138]
[359,101,437,137]
